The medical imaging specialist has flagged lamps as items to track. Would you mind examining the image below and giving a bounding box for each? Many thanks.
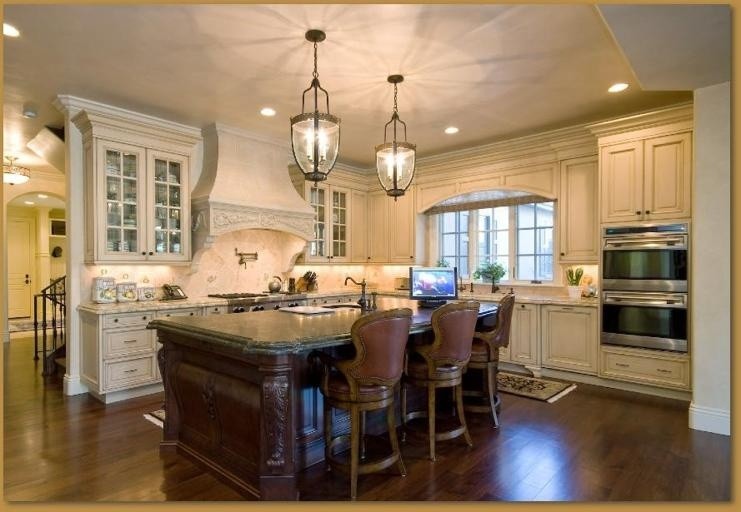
[373,69,414,201]
[23,110,37,119]
[2,155,30,186]
[287,27,340,205]
[27,125,64,174]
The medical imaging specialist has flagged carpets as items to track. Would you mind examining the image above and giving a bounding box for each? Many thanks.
[9,319,65,333]
[492,369,579,404]
[143,403,168,428]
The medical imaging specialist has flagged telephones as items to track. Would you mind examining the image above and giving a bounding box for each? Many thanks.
[158,283,188,301]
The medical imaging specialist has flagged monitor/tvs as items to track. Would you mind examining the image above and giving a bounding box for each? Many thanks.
[409,266,458,310]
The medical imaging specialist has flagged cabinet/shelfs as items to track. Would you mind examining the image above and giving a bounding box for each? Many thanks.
[557,147,602,264]
[598,224,691,399]
[542,304,598,384]
[349,188,370,264]
[368,184,416,264]
[307,296,345,306]
[76,307,155,405]
[203,304,227,318]
[497,304,540,371]
[157,308,202,392]
[346,295,371,305]
[586,100,694,222]
[70,109,203,268]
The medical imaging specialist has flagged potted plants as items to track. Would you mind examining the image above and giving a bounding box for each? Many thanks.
[565,269,584,301]
[474,262,504,293]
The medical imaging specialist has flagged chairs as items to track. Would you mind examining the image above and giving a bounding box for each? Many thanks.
[307,306,411,500]
[402,301,475,461]
[452,290,516,429]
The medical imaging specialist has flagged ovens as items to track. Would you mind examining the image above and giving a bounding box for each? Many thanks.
[598,221,691,354]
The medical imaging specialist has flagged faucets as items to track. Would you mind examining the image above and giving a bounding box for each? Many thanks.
[344,277,375,311]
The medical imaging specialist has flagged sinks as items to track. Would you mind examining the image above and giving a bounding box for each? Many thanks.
[320,304,361,312]
